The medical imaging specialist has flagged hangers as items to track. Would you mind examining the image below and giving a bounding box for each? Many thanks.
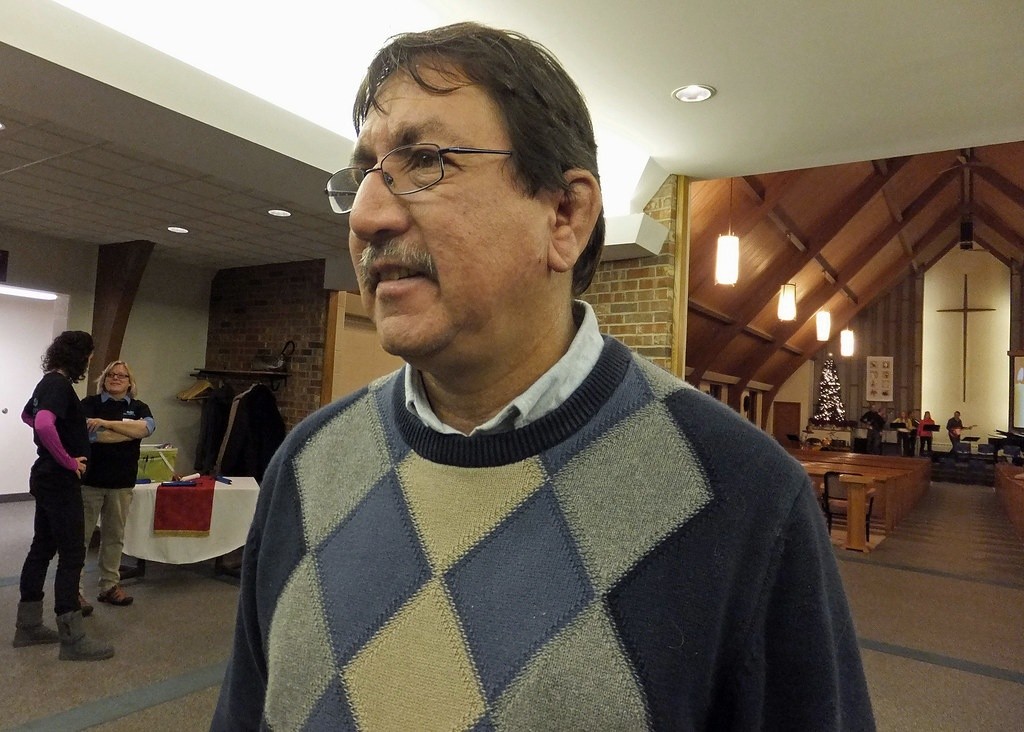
[176,376,214,401]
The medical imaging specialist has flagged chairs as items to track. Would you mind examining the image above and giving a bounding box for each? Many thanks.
[818,471,873,541]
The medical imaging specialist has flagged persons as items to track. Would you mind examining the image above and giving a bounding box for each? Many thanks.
[894,411,935,456]
[212,23,876,732]
[860,404,893,454]
[946,411,972,462]
[80,361,156,613]
[15,332,114,660]
[805,437,832,450]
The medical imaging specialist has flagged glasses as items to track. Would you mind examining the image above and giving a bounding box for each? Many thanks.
[109,372,129,379]
[325,142,512,215]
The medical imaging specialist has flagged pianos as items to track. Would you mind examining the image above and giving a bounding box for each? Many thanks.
[988,426,1024,457]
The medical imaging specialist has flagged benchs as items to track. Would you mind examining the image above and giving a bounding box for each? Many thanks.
[995,463,1024,541]
[786,449,932,537]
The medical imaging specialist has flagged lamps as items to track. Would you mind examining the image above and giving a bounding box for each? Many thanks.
[778,231,797,322]
[840,294,855,357]
[714,178,738,287]
[815,270,831,341]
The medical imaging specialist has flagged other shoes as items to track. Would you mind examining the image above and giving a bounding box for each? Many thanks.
[78,596,94,615]
[97,585,134,607]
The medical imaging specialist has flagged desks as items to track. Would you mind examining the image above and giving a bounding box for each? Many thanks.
[96,477,261,580]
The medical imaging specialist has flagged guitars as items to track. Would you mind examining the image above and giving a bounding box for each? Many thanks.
[950,424,978,438]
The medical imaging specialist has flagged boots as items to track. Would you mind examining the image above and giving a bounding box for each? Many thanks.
[56,612,116,660]
[13,601,59,647]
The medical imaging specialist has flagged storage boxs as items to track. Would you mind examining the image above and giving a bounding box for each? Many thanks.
[137,444,177,480]
